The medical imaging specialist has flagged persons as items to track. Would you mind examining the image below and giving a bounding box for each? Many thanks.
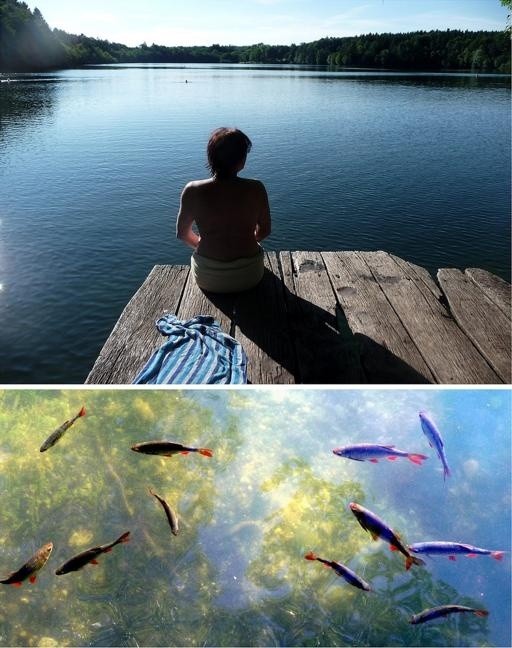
[176,127,271,293]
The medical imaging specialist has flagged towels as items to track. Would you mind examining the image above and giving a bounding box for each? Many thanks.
[132,308,248,384]
